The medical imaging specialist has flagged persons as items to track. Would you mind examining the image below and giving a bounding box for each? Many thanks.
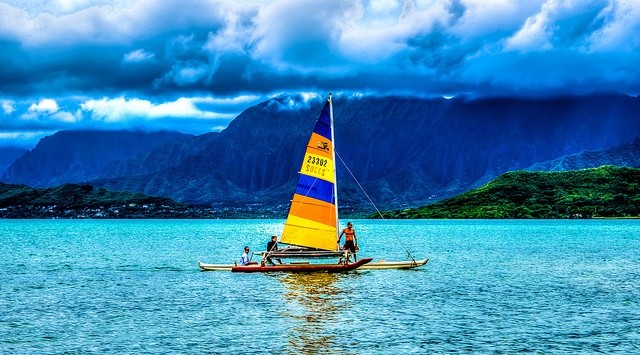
[337,222,359,262]
[267,236,283,266]
[240,246,258,266]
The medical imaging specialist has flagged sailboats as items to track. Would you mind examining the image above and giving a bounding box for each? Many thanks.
[199,93,428,271]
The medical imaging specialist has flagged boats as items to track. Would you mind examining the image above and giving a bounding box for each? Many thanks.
[254,244,345,257]
[232,257,373,269]
